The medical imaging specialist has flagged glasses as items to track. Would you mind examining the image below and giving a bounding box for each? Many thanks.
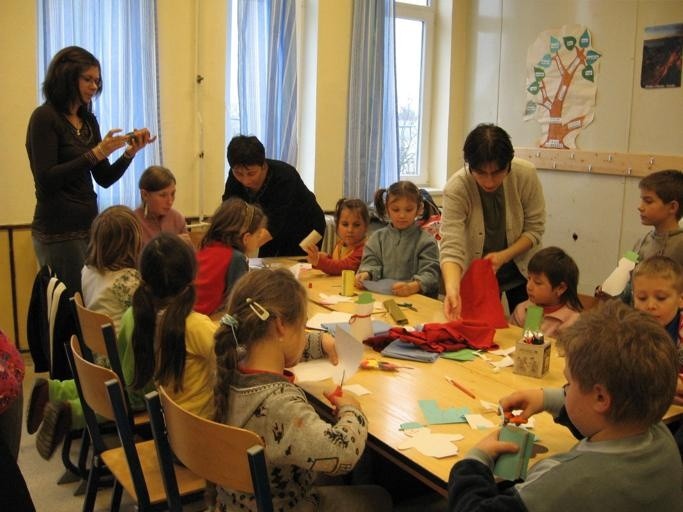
[82,73,102,88]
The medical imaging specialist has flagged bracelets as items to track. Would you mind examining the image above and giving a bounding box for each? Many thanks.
[124,147,135,159]
[97,142,109,160]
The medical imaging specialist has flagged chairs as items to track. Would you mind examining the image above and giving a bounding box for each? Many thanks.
[69,292,149,498]
[63,334,208,511]
[146,385,272,512]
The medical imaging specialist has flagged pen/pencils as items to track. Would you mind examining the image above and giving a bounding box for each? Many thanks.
[522,328,545,344]
[445,375,477,400]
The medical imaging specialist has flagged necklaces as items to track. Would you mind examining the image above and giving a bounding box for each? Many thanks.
[66,113,81,137]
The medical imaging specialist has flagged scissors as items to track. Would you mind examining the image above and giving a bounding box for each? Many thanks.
[398,304,418,312]
[329,370,346,412]
[503,409,528,427]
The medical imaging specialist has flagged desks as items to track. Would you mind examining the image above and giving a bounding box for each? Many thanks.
[244,255,682,499]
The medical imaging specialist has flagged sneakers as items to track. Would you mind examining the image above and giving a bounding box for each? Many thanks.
[35,401,70,460]
[25,377,48,434]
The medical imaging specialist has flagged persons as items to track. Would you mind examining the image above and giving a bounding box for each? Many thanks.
[221,133,326,259]
[436,122,546,326]
[130,162,197,259]
[24,196,268,461]
[507,242,582,345]
[627,255,683,410]
[304,194,368,277]
[352,180,443,296]
[203,266,394,511]
[23,46,158,307]
[446,297,683,511]
[592,167,683,309]
[0,330,27,463]
[129,232,220,472]
[79,204,158,417]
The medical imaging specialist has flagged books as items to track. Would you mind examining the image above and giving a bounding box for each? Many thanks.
[319,318,392,344]
[380,324,441,365]
